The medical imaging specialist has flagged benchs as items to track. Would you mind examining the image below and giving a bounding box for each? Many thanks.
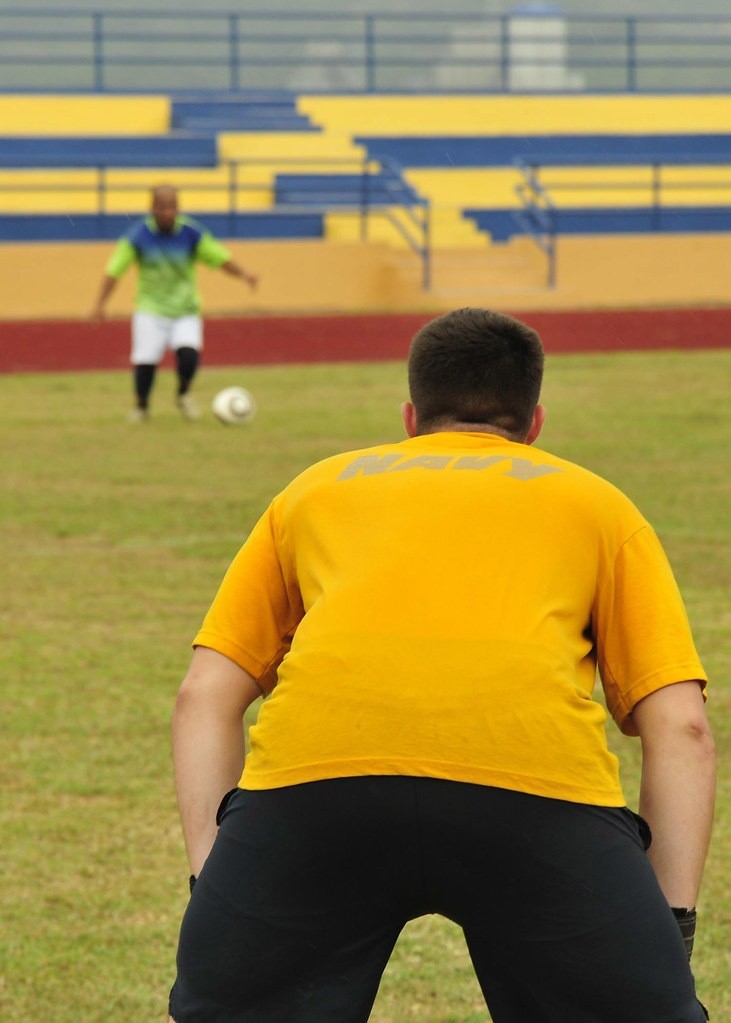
[0,83,731,245]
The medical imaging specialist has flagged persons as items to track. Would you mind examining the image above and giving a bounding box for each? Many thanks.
[169,308,712,1022]
[93,187,258,426]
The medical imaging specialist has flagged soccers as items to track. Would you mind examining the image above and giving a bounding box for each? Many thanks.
[211,385,257,429]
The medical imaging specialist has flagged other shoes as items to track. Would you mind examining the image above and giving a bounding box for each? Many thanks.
[172,392,202,422]
[133,407,150,425]
